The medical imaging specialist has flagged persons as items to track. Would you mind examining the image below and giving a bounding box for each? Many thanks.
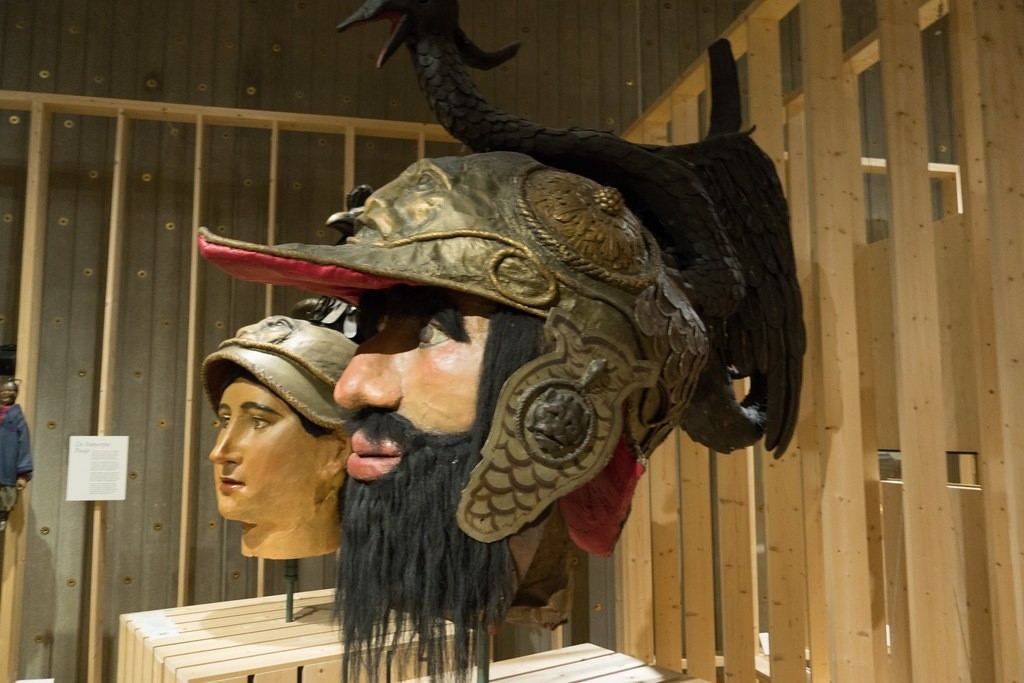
[196,152,708,683]
[200,315,360,560]
[0,379,33,534]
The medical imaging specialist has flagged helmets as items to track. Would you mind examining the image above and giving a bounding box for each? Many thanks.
[198,149,714,547]
